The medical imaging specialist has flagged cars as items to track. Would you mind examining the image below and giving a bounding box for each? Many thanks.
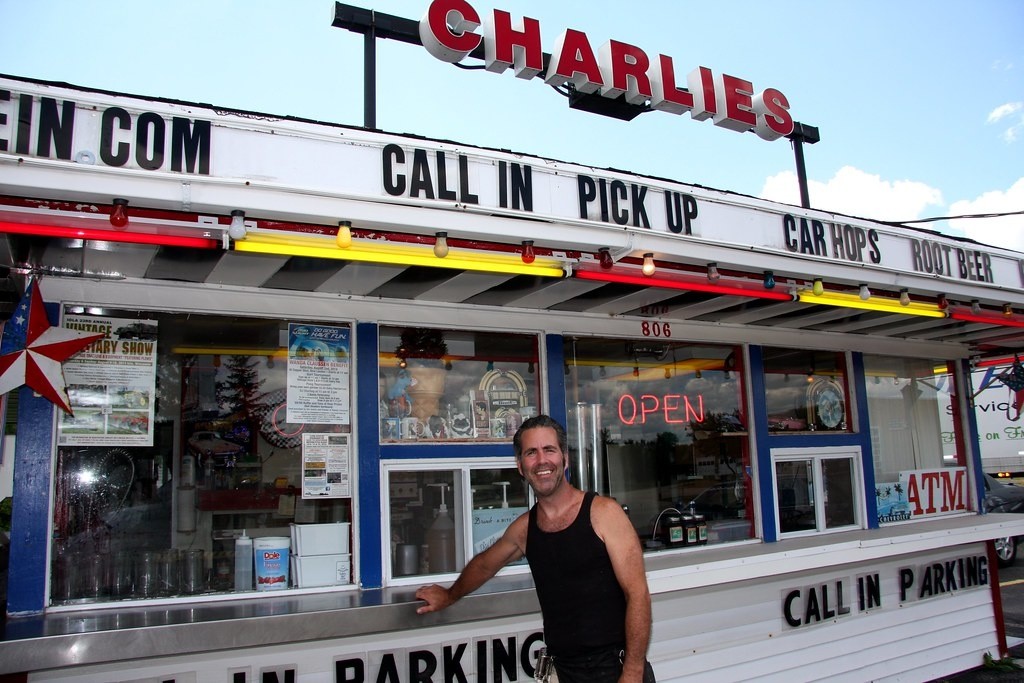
[769,472,853,530]
[947,464,1024,569]
[660,480,747,523]
[184,431,243,468]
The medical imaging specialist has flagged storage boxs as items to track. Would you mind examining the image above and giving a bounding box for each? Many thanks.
[704,519,752,543]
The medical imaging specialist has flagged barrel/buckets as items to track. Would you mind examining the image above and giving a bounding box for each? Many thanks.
[254,537,291,592]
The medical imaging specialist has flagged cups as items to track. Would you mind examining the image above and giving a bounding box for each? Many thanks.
[51,547,204,602]
[489,417,506,439]
[381,395,425,441]
[395,543,420,576]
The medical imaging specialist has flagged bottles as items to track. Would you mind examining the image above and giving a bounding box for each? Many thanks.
[664,515,709,548]
[203,454,238,491]
[234,528,254,594]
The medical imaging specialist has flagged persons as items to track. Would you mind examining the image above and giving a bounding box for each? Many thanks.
[417,415,656,683]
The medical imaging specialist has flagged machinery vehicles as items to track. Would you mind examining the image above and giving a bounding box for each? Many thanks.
[117,389,145,408]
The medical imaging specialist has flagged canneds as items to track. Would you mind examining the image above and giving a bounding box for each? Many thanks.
[663,514,707,547]
